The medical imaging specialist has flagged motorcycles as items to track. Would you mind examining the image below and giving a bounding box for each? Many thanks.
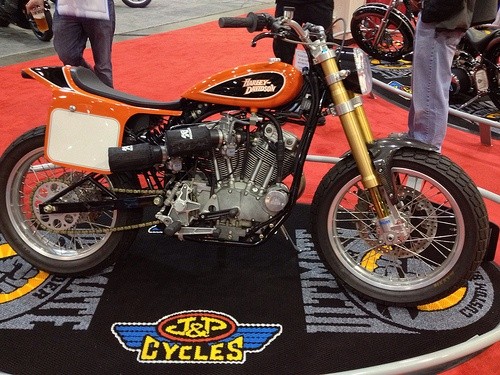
[0,0,53,42]
[349,0,500,109]
[0,7,490,306]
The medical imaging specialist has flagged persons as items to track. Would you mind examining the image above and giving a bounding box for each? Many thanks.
[273,0,336,126]
[25,0,116,91]
[406,1,500,156]
